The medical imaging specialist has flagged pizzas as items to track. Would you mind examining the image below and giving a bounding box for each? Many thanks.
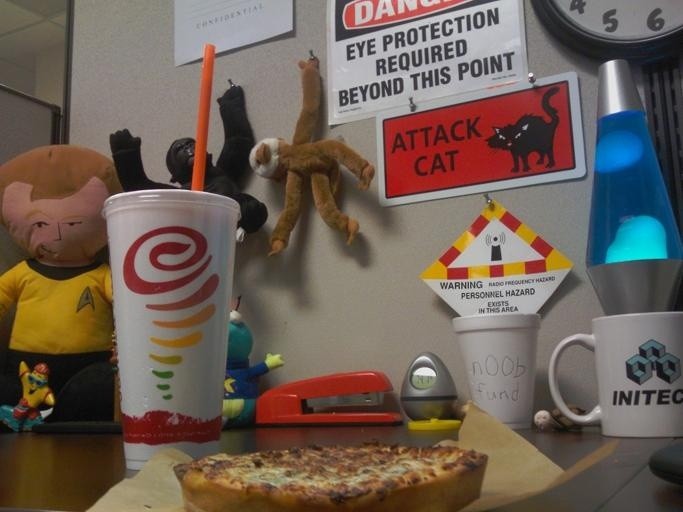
[173,438,489,511]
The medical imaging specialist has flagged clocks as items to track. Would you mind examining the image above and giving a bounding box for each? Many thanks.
[529,0,683,68]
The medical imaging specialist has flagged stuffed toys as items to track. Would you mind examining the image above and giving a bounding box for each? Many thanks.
[0,361,57,432]
[220,310,284,432]
[0,142,123,422]
[247,58,375,256]
[106,85,268,244]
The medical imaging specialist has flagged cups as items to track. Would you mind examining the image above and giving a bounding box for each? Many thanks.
[450,311,542,431]
[97,187,244,471]
[547,310,683,439]
